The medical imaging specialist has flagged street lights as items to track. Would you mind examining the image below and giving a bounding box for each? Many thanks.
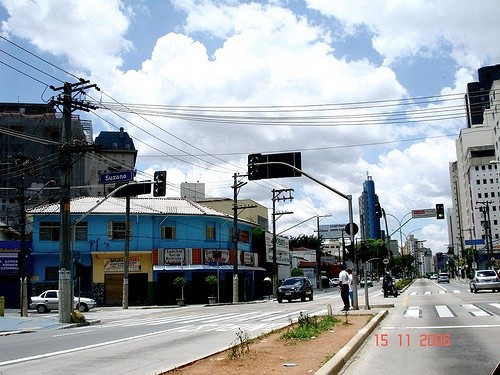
[19,177,56,315]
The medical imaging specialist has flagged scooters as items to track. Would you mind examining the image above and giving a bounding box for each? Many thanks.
[381,280,398,298]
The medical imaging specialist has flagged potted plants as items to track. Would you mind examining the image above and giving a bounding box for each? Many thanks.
[173,277,187,305]
[205,275,218,303]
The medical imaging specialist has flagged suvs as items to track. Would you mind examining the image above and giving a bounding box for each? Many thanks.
[428,272,450,283]
[277,276,314,303]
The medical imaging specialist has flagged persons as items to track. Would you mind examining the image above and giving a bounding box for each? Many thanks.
[338,264,349,312]
[382,272,397,298]
[347,268,355,310]
[263,276,272,296]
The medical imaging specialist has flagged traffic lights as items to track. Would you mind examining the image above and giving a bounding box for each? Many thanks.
[436,204,444,219]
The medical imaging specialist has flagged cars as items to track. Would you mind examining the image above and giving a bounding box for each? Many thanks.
[328,278,340,287]
[360,276,373,287]
[470,269,500,293]
[29,290,97,312]
